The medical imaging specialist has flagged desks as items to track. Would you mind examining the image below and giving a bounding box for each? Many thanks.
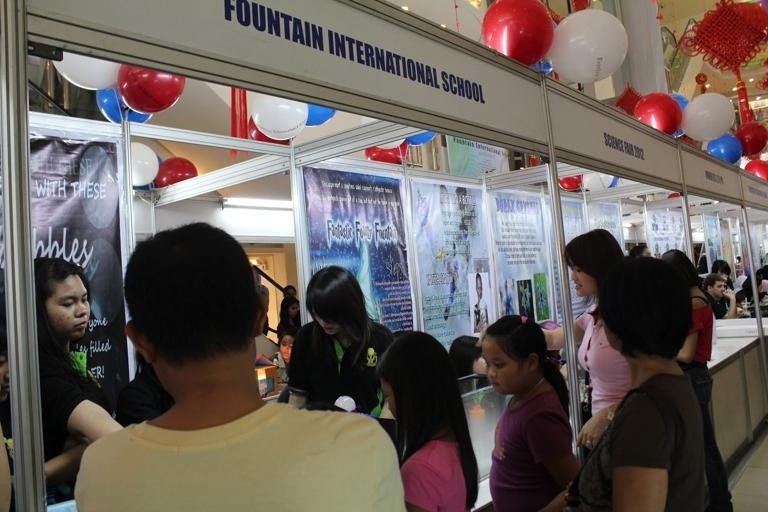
[707,318,767,477]
[47,387,512,511]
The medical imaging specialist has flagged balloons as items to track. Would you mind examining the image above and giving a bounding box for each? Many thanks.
[667,192,680,199]
[478,0,630,85]
[559,170,620,191]
[632,90,768,185]
[130,141,199,192]
[245,90,337,148]
[360,115,439,164]
[51,51,187,123]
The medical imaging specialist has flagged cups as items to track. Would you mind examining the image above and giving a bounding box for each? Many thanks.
[741,302,752,317]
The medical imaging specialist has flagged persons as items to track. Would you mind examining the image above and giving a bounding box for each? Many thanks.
[734,253,768,317]
[251,226,734,512]
[1,249,175,511]
[73,219,408,511]
